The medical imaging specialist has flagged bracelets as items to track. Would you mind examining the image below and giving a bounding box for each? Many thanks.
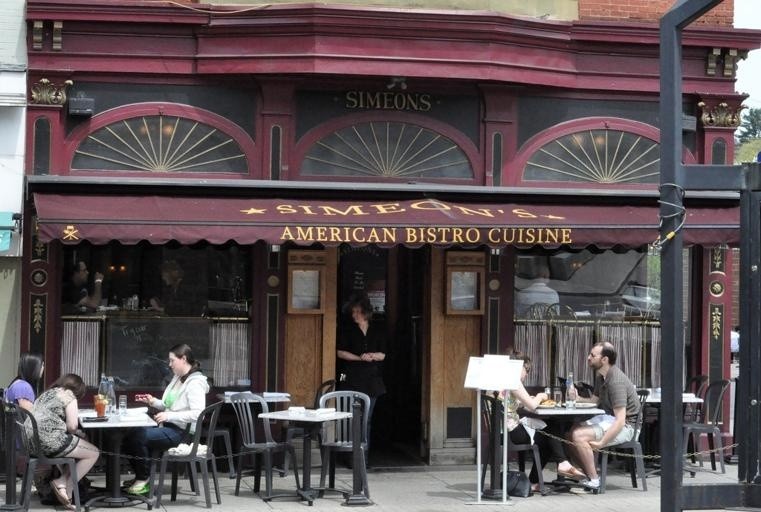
[93,278,103,284]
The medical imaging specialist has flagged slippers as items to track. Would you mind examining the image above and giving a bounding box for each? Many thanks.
[557,466,586,479]
[126,483,149,494]
[122,478,135,486]
[531,484,540,492]
[48,480,69,505]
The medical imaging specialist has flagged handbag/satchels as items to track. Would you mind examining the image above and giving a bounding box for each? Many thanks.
[499,470,533,497]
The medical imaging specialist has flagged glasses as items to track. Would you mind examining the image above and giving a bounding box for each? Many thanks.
[523,365,530,374]
[587,352,602,358]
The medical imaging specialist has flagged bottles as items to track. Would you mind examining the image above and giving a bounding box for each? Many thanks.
[98,372,108,398]
[565,371,578,410]
[106,376,116,413]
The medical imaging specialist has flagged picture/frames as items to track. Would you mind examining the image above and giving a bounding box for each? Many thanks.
[286,264,326,315]
[446,266,486,315]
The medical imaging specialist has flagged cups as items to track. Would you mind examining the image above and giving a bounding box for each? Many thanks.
[553,386,562,408]
[96,402,108,419]
[545,387,552,401]
[132,294,139,311]
[94,393,103,410]
[118,402,128,419]
[122,297,131,311]
[118,395,127,408]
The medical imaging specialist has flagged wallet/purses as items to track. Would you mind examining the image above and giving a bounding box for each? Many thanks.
[83,415,109,422]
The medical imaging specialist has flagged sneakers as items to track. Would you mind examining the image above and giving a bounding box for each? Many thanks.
[583,480,600,487]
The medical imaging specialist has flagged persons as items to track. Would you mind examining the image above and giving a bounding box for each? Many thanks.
[730,326,742,363]
[484,352,587,493]
[151,259,195,318]
[572,342,643,490]
[22,374,100,509]
[78,260,89,282]
[61,259,104,314]
[515,265,560,319]
[2,353,90,505]
[337,293,391,469]
[124,344,210,496]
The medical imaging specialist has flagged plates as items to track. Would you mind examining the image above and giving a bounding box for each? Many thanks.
[536,405,555,408]
[563,402,598,408]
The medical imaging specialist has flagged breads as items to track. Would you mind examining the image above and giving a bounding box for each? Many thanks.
[541,399,556,406]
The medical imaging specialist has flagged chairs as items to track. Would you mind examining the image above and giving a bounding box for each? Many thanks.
[515,302,660,327]
[481,375,731,495]
